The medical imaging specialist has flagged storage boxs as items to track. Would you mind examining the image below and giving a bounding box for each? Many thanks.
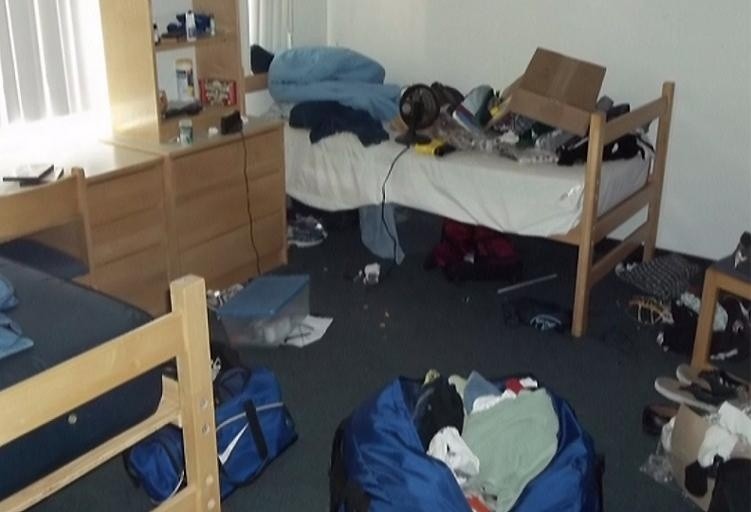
[663,404,751,512]
[499,46,607,138]
[217,272,314,352]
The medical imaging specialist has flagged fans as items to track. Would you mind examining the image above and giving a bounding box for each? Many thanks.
[394,82,442,147]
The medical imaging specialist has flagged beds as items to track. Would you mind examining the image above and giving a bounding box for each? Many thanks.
[1,258,224,511]
[245,70,678,339]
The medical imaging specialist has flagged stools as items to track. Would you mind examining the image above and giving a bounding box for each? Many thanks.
[691,251,751,400]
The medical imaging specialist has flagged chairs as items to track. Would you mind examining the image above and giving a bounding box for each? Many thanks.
[0,167,102,293]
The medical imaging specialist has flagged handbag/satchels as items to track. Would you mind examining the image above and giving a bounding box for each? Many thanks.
[120,367,305,507]
[326,371,605,511]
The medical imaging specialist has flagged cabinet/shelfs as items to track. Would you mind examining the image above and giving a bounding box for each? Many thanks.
[107,111,289,299]
[99,0,248,144]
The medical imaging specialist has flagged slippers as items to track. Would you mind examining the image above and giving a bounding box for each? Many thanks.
[653,360,751,412]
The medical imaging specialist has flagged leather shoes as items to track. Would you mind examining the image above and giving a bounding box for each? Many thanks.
[641,403,680,437]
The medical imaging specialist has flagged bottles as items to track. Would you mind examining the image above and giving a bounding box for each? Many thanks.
[153,24,160,46]
[175,59,198,104]
[179,120,193,144]
[210,13,216,37]
[186,11,198,44]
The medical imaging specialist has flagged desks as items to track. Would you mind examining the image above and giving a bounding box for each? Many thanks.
[0,134,179,317]
